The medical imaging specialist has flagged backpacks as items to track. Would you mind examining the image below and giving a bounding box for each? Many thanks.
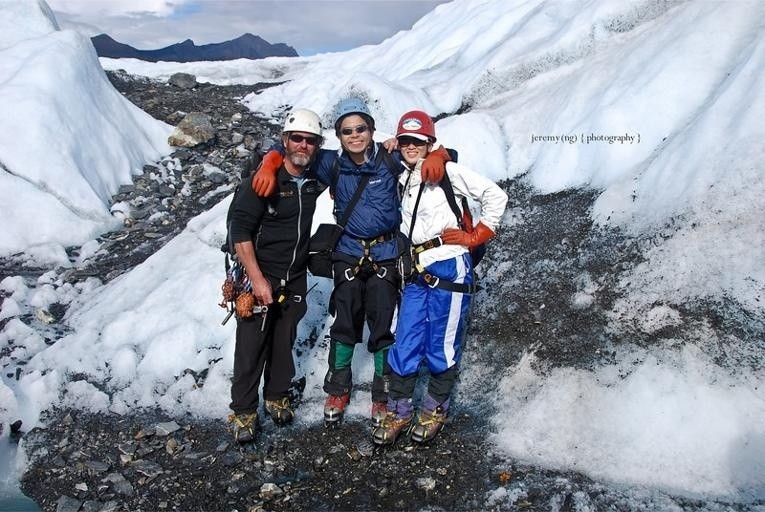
[221,150,280,259]
[437,147,489,269]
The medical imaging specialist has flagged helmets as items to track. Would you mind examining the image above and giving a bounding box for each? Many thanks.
[329,100,378,129]
[395,110,438,142]
[281,107,326,136]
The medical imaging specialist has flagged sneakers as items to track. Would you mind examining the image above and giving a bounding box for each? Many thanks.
[322,390,352,423]
[263,399,296,425]
[231,414,257,444]
[371,399,388,424]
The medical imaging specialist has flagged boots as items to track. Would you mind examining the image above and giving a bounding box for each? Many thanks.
[369,396,415,446]
[410,391,452,444]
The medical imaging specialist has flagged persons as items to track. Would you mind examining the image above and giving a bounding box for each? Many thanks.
[371,111,512,446]
[227,108,400,444]
[252,98,458,423]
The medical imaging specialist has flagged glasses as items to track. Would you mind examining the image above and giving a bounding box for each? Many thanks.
[336,124,370,135]
[285,134,318,145]
[399,138,431,147]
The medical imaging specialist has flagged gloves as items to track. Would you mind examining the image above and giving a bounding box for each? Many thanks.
[442,220,497,247]
[252,148,286,197]
[420,143,454,188]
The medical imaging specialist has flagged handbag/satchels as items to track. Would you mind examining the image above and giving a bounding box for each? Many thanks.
[307,219,342,280]
